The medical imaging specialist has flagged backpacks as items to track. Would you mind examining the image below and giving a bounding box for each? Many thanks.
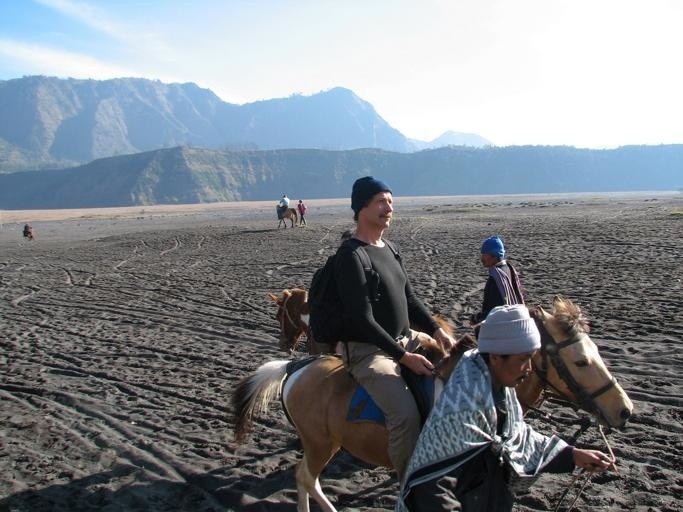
[309,239,403,342]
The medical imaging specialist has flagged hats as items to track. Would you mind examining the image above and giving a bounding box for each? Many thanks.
[351,176,391,212]
[478,303,541,355]
[481,235,505,258]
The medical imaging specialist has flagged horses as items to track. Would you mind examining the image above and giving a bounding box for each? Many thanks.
[22,229,34,240]
[268,287,454,354]
[232,295,636,512]
[275,204,297,228]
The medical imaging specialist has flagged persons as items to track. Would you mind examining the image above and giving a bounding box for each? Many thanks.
[23,223,32,239]
[278,194,289,214]
[333,176,454,489]
[297,200,306,227]
[393,303,615,512]
[469,236,526,326]
